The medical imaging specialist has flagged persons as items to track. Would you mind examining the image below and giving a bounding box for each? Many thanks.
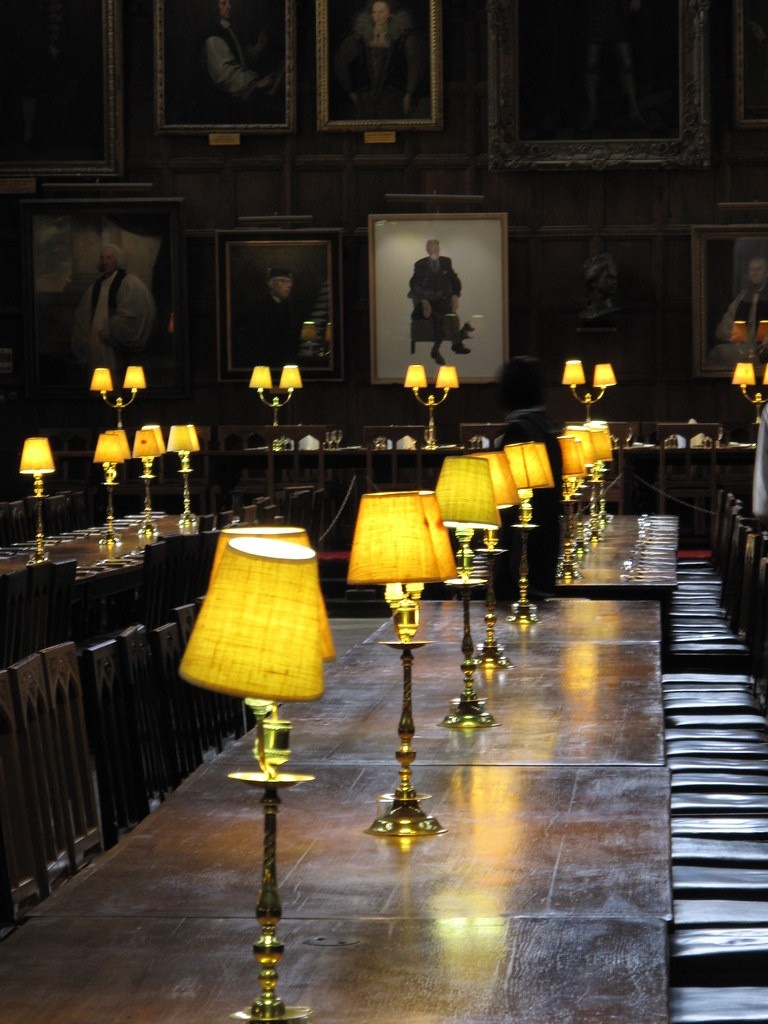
[466,355,564,601]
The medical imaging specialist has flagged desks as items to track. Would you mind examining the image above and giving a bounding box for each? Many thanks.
[0,512,211,621]
[445,514,680,599]
[242,438,515,502]
[557,443,757,517]
[0,763,669,1023]
[206,597,664,768]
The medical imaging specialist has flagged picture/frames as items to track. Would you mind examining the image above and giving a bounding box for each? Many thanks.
[17,197,193,404]
[151,1,295,136]
[368,213,511,385]
[732,0,768,130]
[485,1,712,176]
[315,1,445,130]
[1,1,125,178]
[689,223,768,379]
[212,228,343,384]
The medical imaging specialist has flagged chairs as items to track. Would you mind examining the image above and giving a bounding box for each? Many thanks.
[0,421,325,929]
[654,417,768,1024]
[364,421,424,498]
[1,485,328,933]
[461,421,516,450]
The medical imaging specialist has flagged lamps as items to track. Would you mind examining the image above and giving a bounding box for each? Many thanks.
[219,527,336,768]
[84,365,147,428]
[560,360,619,416]
[345,440,557,847]
[20,437,55,567]
[92,434,123,546]
[179,538,324,1024]
[403,364,461,447]
[730,362,768,417]
[110,430,130,462]
[246,362,301,425]
[168,425,201,525]
[561,417,613,579]
[144,425,166,457]
[134,430,161,536]
